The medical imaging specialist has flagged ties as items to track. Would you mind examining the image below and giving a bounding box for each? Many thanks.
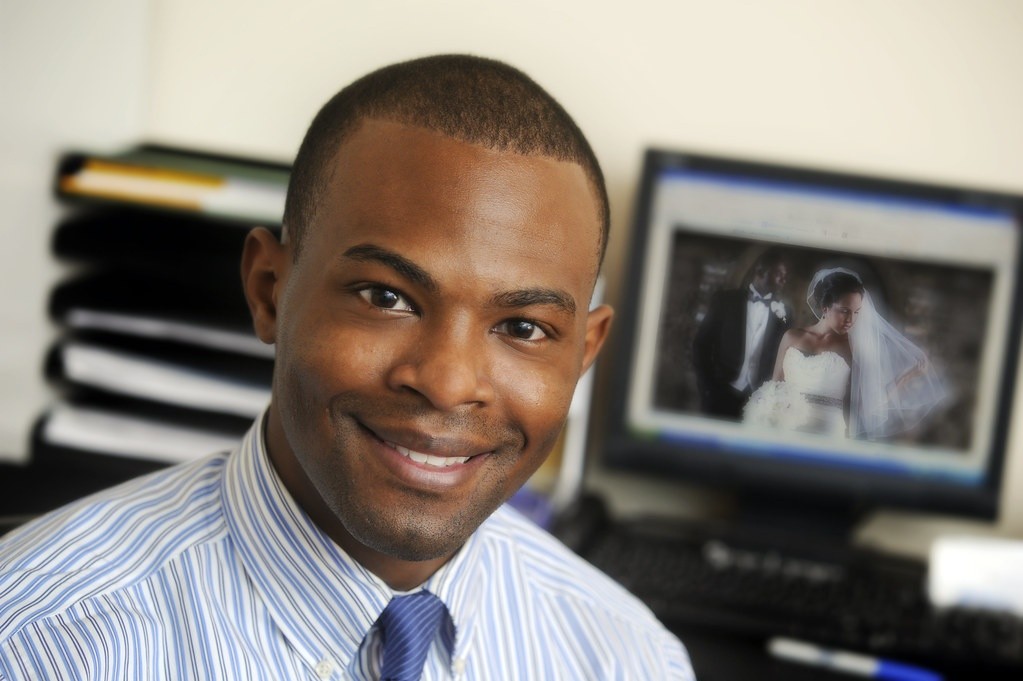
[379,588,445,681]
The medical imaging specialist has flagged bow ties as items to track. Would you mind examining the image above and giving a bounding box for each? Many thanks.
[753,295,771,307]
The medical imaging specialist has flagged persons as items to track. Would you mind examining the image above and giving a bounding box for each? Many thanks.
[742,265,946,438]
[691,252,794,418]
[0,52,702,681]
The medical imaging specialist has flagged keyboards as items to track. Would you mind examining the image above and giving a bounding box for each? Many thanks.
[573,530,927,658]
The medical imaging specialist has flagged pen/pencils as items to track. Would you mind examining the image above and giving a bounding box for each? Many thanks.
[768,637,943,681]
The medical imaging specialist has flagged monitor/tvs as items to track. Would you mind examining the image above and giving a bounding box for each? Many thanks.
[588,144,1023,571]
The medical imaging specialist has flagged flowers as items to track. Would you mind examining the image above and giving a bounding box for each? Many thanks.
[739,378,813,432]
[769,301,787,325]
